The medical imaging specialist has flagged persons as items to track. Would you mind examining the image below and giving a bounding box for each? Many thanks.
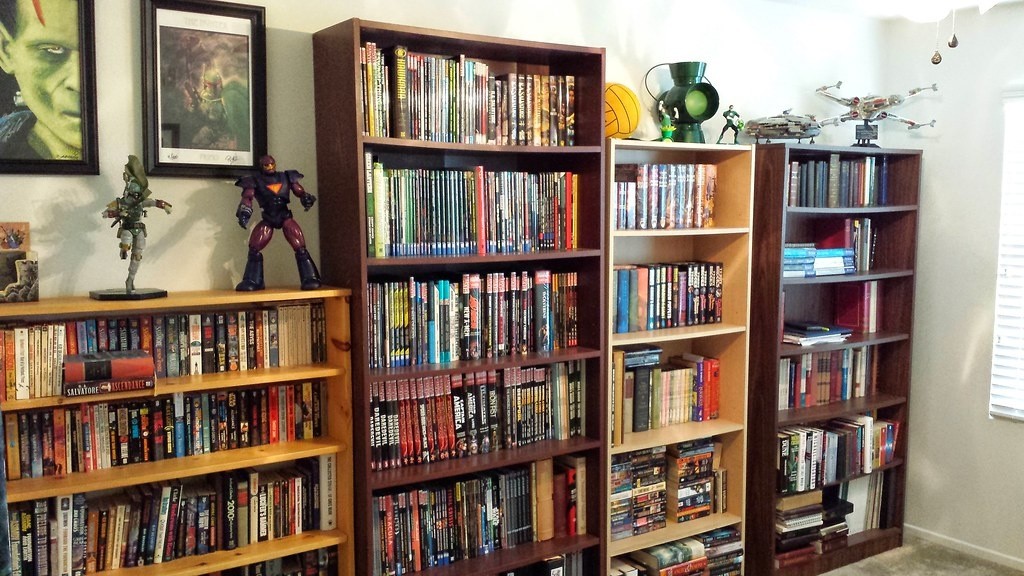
[716,105,741,144]
[100,154,174,293]
[0,0,84,161]
[234,153,324,291]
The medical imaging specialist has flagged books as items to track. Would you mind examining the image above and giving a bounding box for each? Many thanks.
[0,297,328,403]
[781,216,878,278]
[198,544,338,576]
[4,377,329,481]
[616,162,718,228]
[497,547,583,576]
[365,268,579,368]
[370,449,586,576]
[612,258,722,334]
[370,355,586,472]
[609,525,743,576]
[360,40,576,146]
[608,435,728,541]
[362,151,578,259]
[9,452,337,576]
[776,408,901,496]
[612,344,721,448]
[787,151,889,208]
[772,471,885,568]
[777,343,878,410]
[780,280,884,350]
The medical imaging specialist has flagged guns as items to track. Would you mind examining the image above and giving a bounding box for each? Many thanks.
[111,197,145,228]
[183,80,202,109]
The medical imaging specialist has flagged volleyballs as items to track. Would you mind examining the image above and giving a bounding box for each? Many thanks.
[605,81,641,139]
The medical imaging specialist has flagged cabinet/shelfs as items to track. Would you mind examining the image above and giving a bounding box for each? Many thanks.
[744,141,925,576]
[606,134,756,576]
[1,280,357,576]
[311,17,608,576]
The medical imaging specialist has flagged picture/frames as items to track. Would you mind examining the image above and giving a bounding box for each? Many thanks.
[140,0,268,179]
[0,0,99,176]
